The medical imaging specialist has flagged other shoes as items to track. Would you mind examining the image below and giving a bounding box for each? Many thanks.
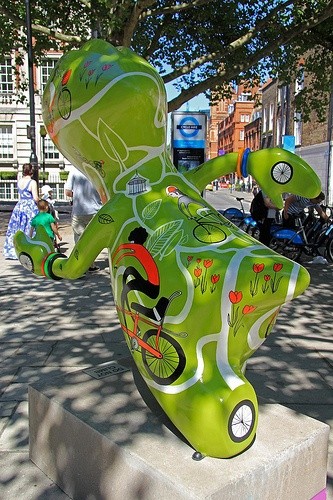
[88,265,100,272]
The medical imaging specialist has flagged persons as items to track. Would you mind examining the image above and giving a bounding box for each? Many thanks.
[249,187,277,249]
[251,183,260,196]
[64,164,104,279]
[2,163,40,260]
[282,192,331,231]
[40,185,60,221]
[29,199,62,245]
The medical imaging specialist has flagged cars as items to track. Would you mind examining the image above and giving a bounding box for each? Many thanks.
[221,183,227,189]
[205,180,214,191]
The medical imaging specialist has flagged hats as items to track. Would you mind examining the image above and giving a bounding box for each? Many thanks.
[40,185,53,194]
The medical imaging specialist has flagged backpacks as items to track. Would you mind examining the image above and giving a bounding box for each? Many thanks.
[250,190,268,220]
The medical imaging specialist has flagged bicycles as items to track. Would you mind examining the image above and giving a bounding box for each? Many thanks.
[223,197,333,263]
